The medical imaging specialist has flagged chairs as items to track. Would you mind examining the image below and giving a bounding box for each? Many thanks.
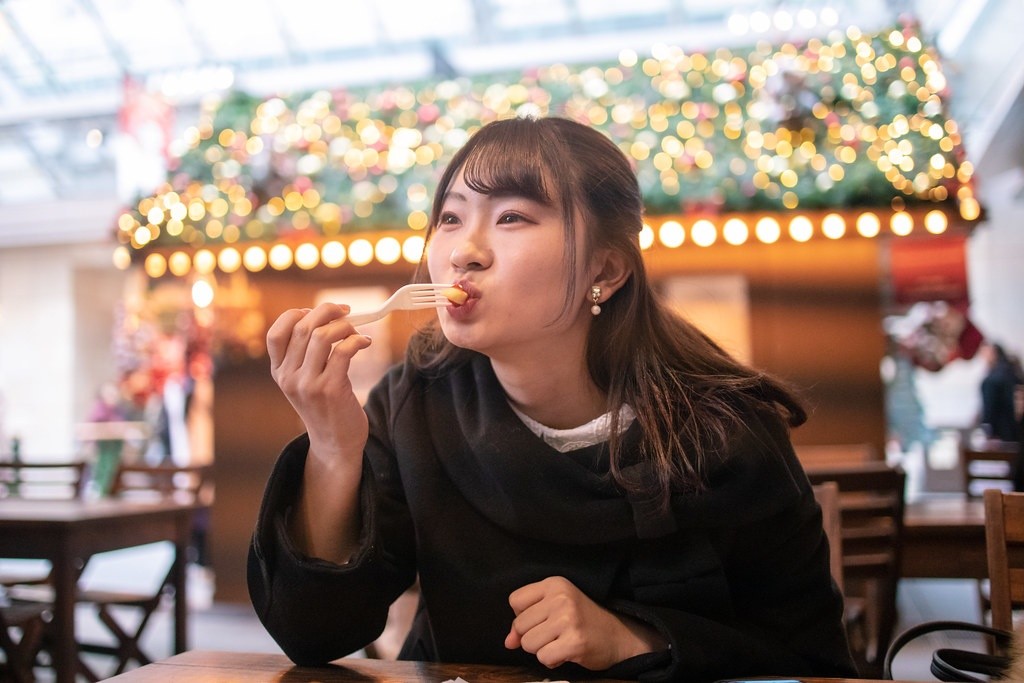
[810,434,1024,680]
[0,459,224,683]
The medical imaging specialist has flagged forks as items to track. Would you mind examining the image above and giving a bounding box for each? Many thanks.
[330,283,454,326]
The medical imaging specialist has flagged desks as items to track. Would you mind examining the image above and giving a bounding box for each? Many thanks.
[0,495,210,683]
[903,496,1023,581]
[106,650,949,683]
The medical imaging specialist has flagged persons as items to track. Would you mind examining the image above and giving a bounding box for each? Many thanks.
[246,118,858,680]
[881,336,926,496]
[978,343,1015,439]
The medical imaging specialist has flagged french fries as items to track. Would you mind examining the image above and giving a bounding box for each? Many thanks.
[441,288,468,306]
